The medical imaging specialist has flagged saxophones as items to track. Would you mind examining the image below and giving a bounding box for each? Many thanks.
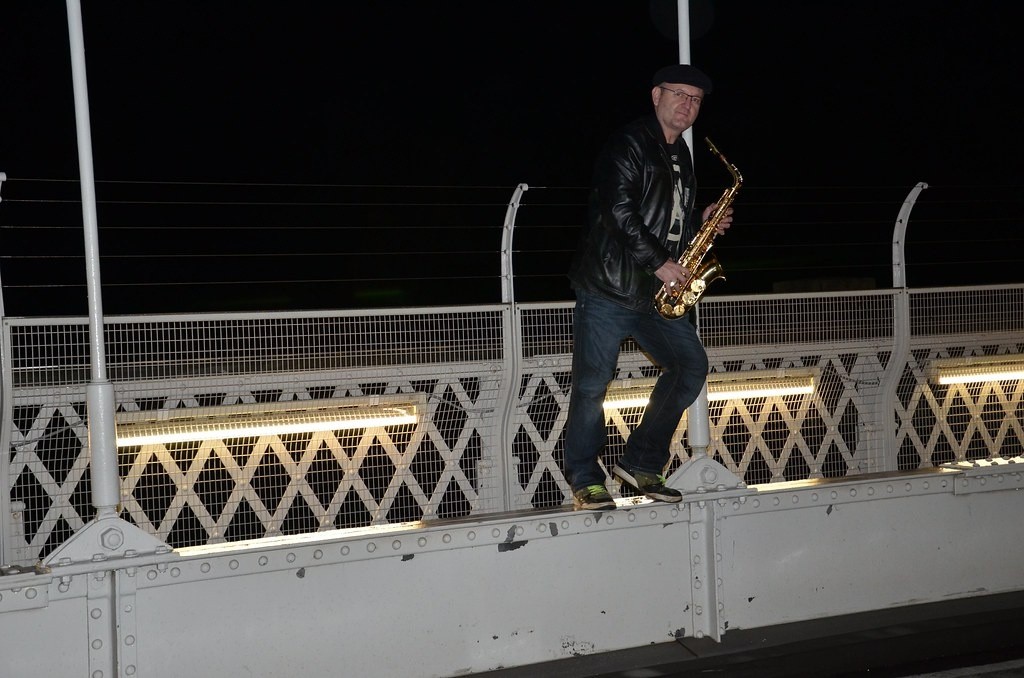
[653,127,744,321]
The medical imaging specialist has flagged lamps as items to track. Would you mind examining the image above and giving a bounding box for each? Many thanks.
[112,393,427,447]
[602,362,821,410]
[927,357,1024,387]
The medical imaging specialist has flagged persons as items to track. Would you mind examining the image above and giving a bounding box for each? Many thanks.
[564,66,736,511]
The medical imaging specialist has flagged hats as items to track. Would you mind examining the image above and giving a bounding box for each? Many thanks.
[652,64,712,95]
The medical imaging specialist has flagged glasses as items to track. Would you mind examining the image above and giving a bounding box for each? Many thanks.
[660,85,702,107]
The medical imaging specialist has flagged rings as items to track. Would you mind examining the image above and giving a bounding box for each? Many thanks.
[671,282,677,288]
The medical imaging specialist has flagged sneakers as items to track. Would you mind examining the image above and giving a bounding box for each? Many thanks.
[613,460,682,502]
[573,485,616,509]
